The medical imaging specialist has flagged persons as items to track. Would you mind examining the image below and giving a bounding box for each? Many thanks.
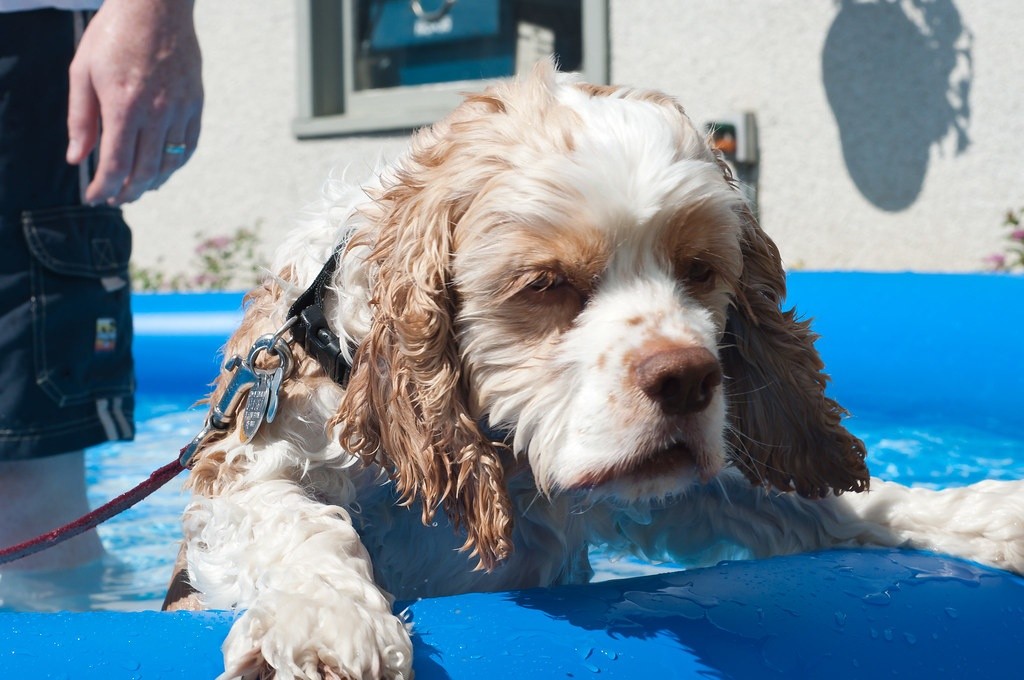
[0,0,205,573]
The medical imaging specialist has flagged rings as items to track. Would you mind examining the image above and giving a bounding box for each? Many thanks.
[164,143,186,154]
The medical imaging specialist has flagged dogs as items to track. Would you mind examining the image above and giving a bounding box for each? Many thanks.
[181,58,1024,680]
